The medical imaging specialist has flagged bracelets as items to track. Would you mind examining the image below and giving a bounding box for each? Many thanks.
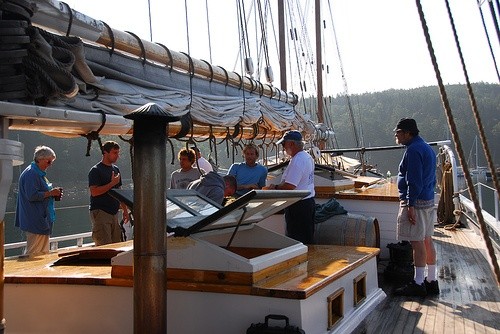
[275,184,278,190]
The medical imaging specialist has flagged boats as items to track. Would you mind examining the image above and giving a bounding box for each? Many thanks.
[457,130,500,182]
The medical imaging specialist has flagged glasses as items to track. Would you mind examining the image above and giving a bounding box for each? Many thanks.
[40,159,52,164]
[397,130,407,134]
[282,141,285,147]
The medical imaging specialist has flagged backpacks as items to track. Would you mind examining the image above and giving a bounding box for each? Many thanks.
[384,240,415,282]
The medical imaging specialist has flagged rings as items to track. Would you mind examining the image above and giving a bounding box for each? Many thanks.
[410,219,412,220]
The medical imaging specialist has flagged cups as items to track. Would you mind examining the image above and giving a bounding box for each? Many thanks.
[55,187,63,201]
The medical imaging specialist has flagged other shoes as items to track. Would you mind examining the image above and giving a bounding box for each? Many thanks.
[394,279,427,297]
[420,277,440,295]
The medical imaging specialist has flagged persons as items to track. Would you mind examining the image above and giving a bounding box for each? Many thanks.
[169,148,207,194]
[262,130,316,249]
[190,147,214,173]
[391,118,444,295]
[88,140,130,248]
[14,145,65,256]
[227,143,268,203]
[186,170,237,209]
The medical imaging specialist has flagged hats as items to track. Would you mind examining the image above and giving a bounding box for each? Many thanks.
[393,118,420,132]
[276,130,302,145]
[191,147,200,152]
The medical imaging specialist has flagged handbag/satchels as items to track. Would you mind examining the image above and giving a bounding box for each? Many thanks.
[314,197,349,224]
[246,314,306,334]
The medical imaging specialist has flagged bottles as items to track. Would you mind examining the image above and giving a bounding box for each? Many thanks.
[387,170,392,183]
